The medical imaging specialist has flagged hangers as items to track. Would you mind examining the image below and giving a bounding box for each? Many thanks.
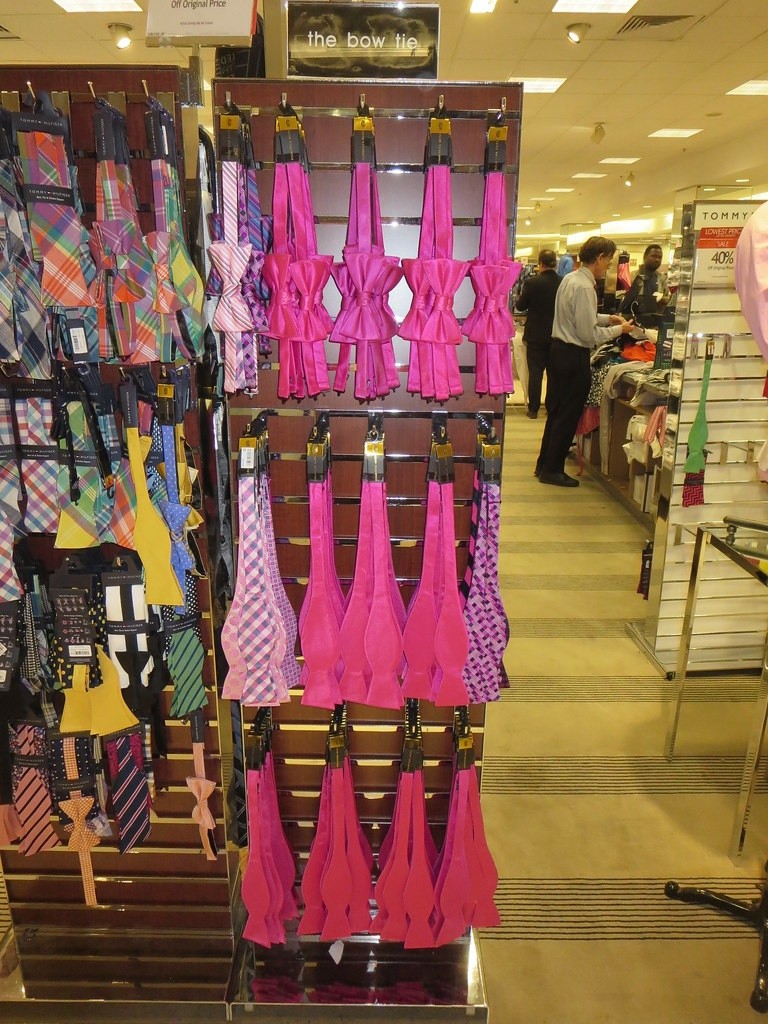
[626,301,645,334]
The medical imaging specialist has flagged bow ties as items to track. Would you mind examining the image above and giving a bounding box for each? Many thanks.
[0,93,524,947]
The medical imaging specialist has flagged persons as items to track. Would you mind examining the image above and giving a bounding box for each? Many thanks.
[515,249,565,419]
[620,244,675,325]
[534,236,635,487]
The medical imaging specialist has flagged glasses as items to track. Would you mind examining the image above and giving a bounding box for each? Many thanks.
[602,256,612,265]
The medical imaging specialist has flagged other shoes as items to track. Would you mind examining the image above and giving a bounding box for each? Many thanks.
[527,412,537,419]
[539,473,579,487]
[535,467,540,475]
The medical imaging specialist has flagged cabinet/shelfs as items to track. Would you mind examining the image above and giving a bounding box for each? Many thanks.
[570,374,669,531]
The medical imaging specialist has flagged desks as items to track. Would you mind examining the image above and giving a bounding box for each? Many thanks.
[663,520,768,867]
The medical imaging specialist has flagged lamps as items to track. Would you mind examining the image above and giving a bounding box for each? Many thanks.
[592,126,607,144]
[567,23,591,44]
[111,26,132,49]
[623,174,636,186]
[535,202,540,212]
